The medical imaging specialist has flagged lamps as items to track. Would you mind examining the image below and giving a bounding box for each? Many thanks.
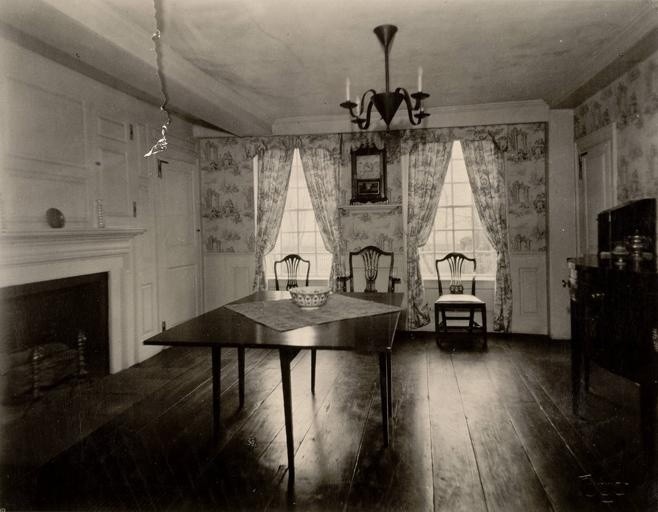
[334,20,431,134]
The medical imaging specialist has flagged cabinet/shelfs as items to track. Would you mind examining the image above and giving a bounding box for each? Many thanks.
[557,245,657,470]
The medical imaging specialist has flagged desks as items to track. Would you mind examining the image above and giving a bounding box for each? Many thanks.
[135,276,411,508]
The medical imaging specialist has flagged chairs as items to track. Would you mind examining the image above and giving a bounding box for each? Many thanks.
[271,253,313,292]
[432,250,489,354]
[332,244,401,296]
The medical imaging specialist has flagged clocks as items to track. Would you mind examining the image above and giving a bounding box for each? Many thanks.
[347,141,390,208]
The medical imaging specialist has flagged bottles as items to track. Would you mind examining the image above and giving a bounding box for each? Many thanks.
[95,199,106,228]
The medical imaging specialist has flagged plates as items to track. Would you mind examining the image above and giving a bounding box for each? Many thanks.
[46,208,65,229]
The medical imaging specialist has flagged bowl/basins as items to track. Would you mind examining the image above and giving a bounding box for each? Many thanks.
[289,285,333,310]
